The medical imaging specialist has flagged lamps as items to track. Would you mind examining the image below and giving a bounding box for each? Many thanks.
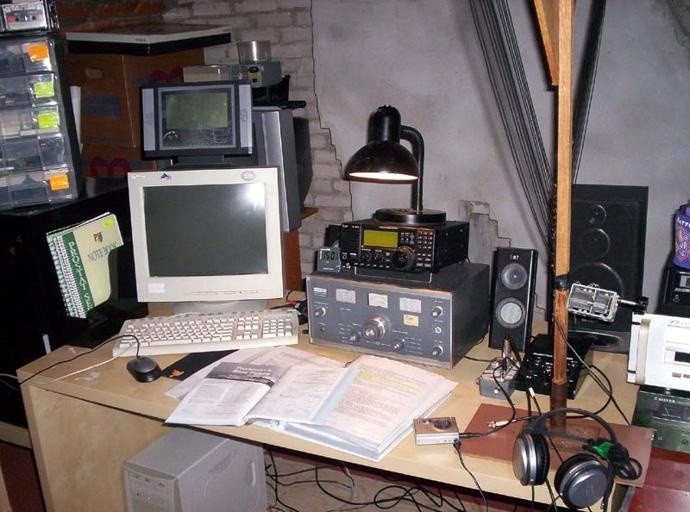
[342,103,449,224]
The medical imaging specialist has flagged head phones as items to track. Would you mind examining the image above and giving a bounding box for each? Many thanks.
[512,407,619,510]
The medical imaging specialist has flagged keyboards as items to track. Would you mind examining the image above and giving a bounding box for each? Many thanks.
[111,310,300,357]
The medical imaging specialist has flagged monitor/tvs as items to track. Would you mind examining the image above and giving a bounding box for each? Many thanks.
[126,164,287,314]
[156,108,314,233]
[139,79,253,165]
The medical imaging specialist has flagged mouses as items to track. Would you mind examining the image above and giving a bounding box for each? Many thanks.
[126,357,162,383]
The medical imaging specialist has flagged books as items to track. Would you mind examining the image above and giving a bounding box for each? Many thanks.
[53,211,126,320]
[44,221,96,318]
[164,342,461,465]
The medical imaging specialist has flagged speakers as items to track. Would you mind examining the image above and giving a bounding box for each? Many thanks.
[488,246,539,353]
[545,183,649,354]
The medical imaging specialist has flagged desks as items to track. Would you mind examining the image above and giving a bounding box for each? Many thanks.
[15,204,639,512]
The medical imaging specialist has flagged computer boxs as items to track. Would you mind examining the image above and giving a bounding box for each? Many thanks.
[120,426,268,512]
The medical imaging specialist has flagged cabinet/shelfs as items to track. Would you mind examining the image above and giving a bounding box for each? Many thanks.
[0,176,148,448]
